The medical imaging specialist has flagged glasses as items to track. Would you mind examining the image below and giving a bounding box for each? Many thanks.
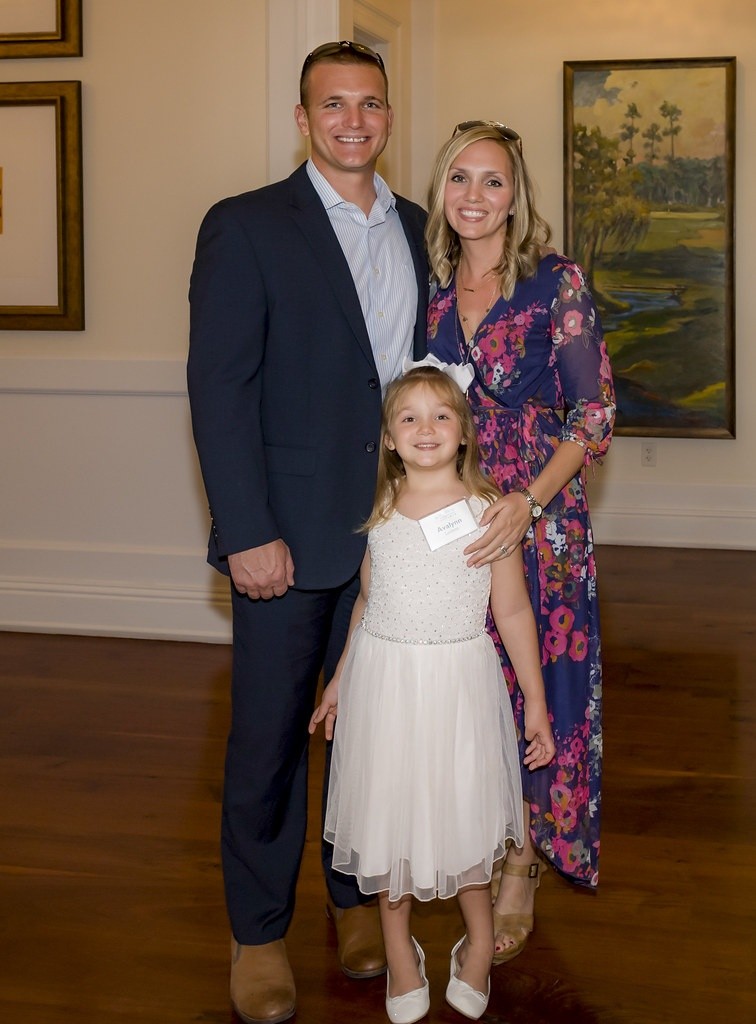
[452,120,523,156]
[300,41,387,102]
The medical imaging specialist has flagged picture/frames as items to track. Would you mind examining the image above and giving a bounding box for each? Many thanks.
[1,82,84,332]
[565,56,736,440]
[0,0,84,58]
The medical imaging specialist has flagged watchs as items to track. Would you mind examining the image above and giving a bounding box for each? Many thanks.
[518,486,543,523]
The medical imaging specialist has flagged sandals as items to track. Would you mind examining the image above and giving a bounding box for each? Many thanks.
[490,838,544,965]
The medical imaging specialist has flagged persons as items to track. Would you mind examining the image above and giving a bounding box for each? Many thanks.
[301,353,558,1022]
[184,40,449,1024]
[411,120,617,962]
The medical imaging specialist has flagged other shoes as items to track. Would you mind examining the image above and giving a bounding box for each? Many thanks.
[386,933,492,1024]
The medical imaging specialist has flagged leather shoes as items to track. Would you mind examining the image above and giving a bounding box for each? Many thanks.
[231,890,389,1023]
